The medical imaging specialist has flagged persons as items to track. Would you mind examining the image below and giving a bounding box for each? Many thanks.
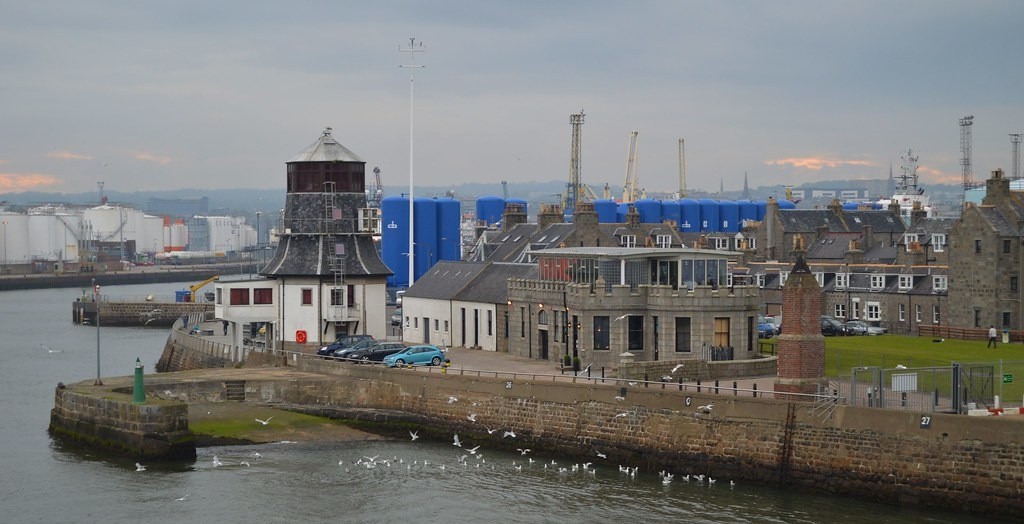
[188,322,199,334]
[223,321,229,335]
[987,325,997,349]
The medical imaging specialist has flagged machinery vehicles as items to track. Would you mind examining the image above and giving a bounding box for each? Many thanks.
[187,275,220,302]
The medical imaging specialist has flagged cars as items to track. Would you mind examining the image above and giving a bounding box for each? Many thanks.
[393,307,402,326]
[383,346,444,368]
[758,314,782,338]
[820,315,883,337]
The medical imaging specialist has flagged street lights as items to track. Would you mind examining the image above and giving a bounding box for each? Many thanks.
[397,36,428,290]
[94,285,103,385]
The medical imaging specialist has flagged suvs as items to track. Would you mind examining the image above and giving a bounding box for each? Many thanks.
[317,335,407,364]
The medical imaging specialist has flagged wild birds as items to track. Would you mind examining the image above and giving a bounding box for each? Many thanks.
[41,344,62,353]
[136,253,735,501]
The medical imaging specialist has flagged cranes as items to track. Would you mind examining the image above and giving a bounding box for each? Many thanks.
[550,103,647,223]
[501,180,510,198]
[373,166,383,196]
[676,137,691,198]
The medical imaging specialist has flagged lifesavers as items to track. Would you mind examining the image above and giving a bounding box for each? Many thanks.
[297,332,305,342]
